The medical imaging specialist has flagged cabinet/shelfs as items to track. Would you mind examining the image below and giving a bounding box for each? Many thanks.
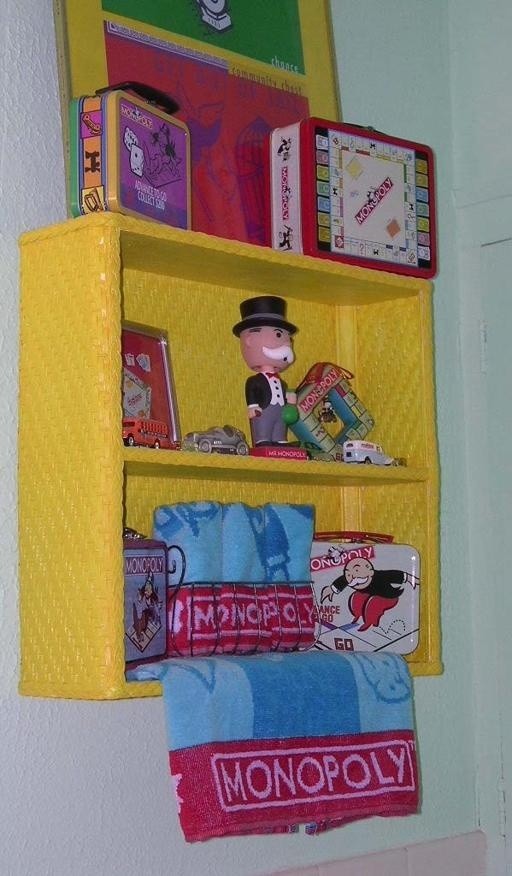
[17,209,445,703]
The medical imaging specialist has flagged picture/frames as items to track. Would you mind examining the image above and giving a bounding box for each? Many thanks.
[120,318,183,449]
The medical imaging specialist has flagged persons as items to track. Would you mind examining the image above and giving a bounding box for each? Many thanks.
[232,295,300,450]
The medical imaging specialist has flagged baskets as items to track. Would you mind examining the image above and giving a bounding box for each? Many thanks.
[166,581,321,657]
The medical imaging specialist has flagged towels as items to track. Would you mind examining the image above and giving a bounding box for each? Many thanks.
[152,500,316,659]
[126,649,422,844]
